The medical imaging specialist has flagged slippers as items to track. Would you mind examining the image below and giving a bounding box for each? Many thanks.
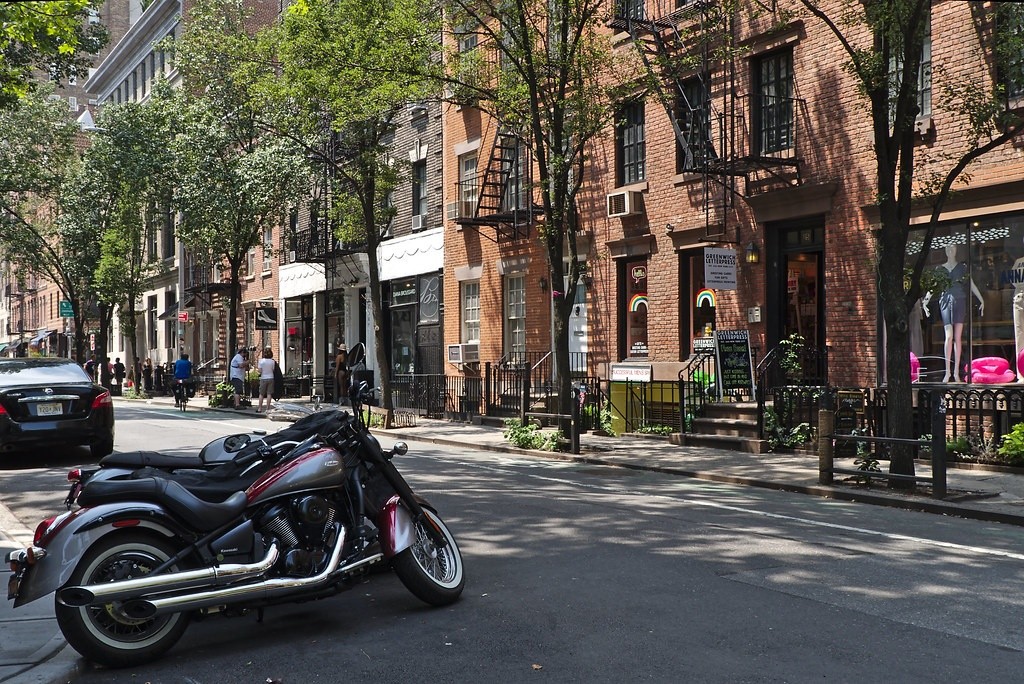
[256,410,261,413]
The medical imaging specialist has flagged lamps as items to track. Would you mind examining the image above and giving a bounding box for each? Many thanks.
[746,242,760,265]
[580,274,592,289]
[539,275,549,294]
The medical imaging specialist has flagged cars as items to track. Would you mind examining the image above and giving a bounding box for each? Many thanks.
[0,356,115,469]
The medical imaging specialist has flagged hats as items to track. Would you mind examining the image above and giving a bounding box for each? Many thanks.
[338,344,350,350]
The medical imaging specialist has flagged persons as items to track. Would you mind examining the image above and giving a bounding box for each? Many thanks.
[334,344,348,405]
[908,244,985,382]
[256,348,275,412]
[1005,255,1024,378]
[172,353,192,406]
[83,356,152,394]
[229,348,252,409]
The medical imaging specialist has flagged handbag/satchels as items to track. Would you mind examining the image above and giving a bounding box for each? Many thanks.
[109,372,114,379]
[122,370,126,377]
[186,382,197,398]
[174,382,182,398]
[128,378,132,386]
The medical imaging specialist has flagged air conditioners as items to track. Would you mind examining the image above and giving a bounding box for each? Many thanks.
[411,215,427,230]
[289,250,297,262]
[261,261,271,272]
[607,191,643,218]
[378,224,394,237]
[448,344,479,364]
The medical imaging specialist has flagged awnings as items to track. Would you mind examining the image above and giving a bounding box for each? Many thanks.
[158,295,195,321]
[0,329,58,353]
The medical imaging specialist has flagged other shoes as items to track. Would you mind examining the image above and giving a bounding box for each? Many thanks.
[175,404,179,406]
[234,405,246,410]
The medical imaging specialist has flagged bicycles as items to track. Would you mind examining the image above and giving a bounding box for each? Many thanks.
[174,379,188,412]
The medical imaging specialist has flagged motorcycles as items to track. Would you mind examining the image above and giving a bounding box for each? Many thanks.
[4,341,468,669]
